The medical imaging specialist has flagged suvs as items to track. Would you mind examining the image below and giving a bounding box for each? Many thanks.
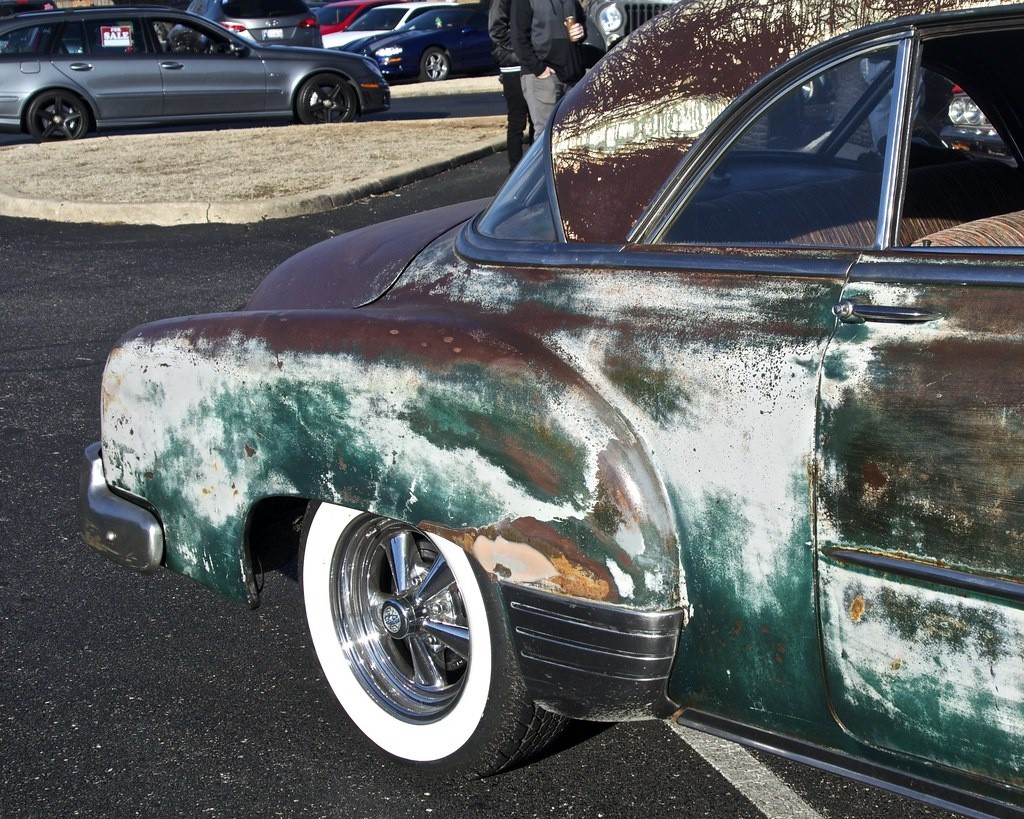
[165,0,323,57]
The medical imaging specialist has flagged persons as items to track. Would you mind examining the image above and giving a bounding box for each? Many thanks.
[487,0,588,174]
[865,58,925,151]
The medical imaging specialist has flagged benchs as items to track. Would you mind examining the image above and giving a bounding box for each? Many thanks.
[669,160,1024,250]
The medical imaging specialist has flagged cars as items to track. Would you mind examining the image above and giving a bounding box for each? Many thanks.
[0,4,391,144]
[0,0,142,57]
[336,3,498,83]
[76,0,1024,819]
[322,1,459,49]
[312,0,413,38]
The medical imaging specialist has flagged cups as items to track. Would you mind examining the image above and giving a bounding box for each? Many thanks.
[565,16,579,43]
